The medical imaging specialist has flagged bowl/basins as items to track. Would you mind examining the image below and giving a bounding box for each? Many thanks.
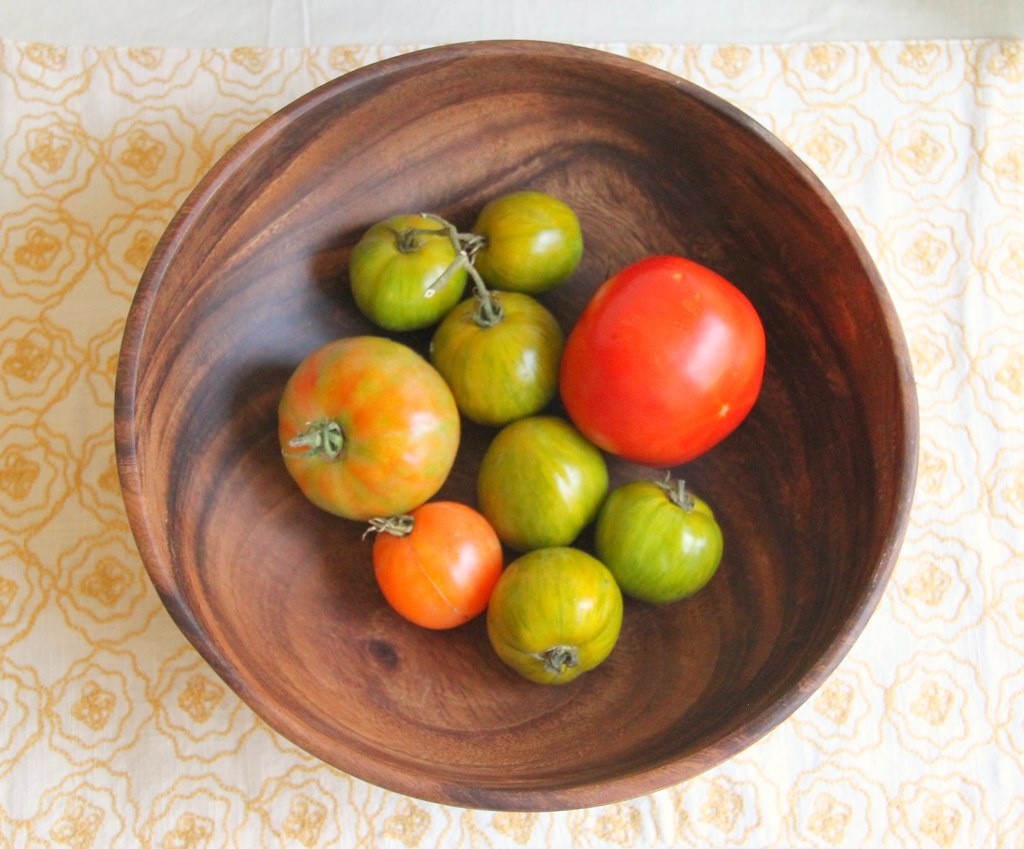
[113,39,920,812]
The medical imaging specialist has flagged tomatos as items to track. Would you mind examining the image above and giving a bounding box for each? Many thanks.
[279,193,765,684]
